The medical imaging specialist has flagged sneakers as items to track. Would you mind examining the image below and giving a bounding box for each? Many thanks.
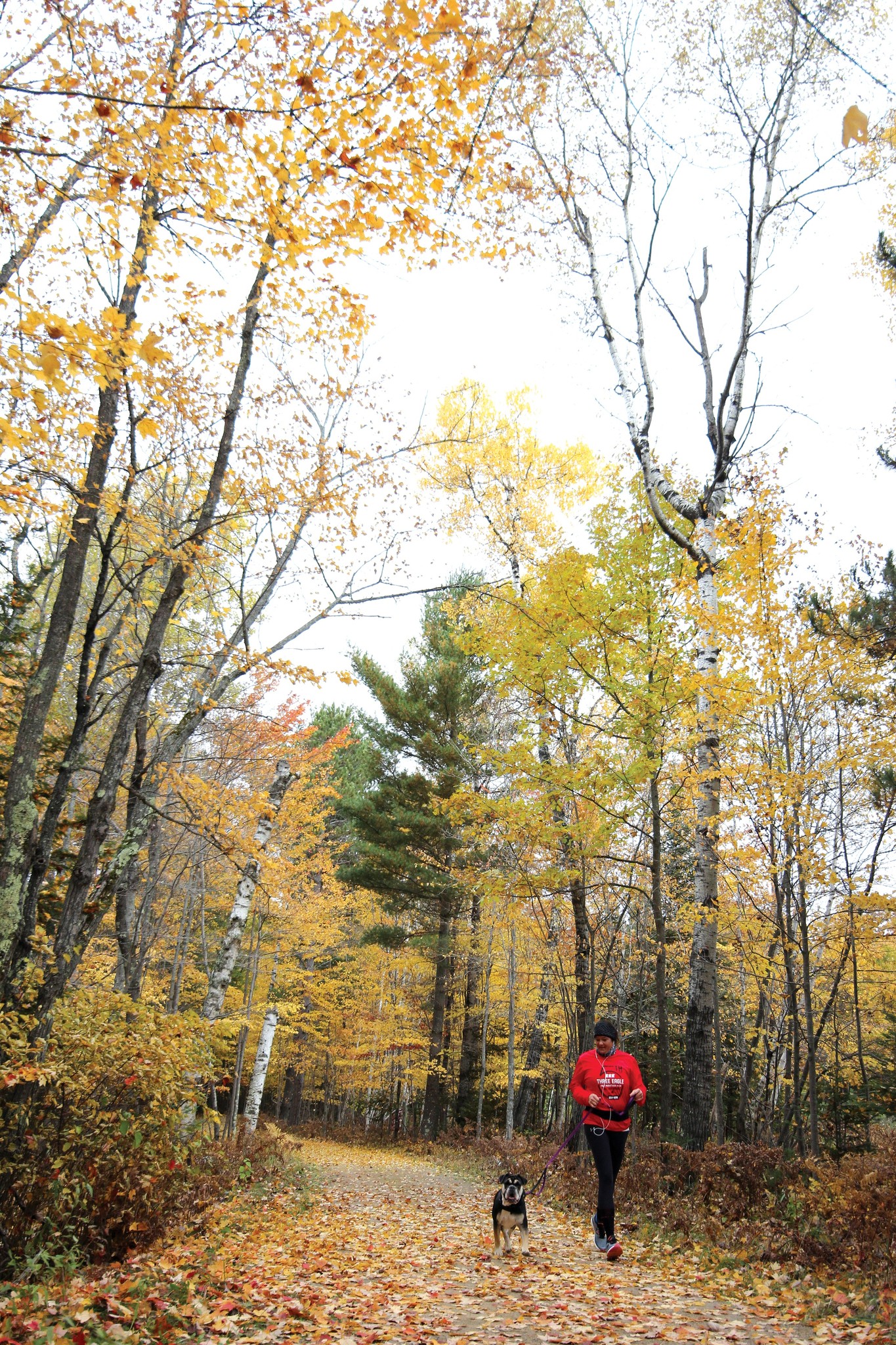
[590,1213,607,1251]
[606,1235,623,1261]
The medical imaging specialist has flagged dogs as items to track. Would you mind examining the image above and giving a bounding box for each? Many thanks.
[491,1173,529,1256]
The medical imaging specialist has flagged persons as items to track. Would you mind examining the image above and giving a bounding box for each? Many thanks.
[569,1020,647,1261]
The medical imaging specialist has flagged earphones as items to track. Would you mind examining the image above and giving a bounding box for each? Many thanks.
[613,1041,614,1044]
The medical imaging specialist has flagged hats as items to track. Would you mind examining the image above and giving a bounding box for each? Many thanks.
[594,1020,617,1042]
